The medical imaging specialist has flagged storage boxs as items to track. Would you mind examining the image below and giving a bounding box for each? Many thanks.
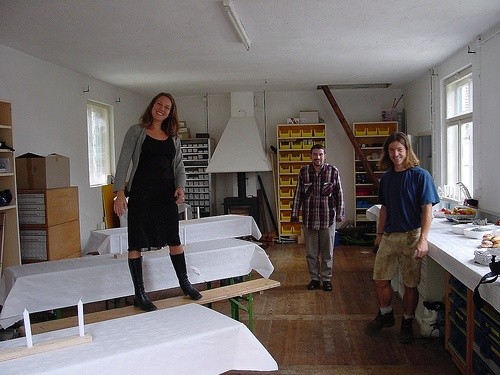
[176,120,186,127]
[366,127,378,135]
[195,132,210,138]
[378,127,389,134]
[20,219,82,261]
[178,127,190,134]
[17,185,79,227]
[355,127,367,135]
[178,132,192,138]
[279,126,325,233]
[180,143,209,213]
[15,148,71,189]
[299,110,320,123]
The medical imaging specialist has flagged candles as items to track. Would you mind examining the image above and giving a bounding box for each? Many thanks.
[22,306,32,348]
[442,184,461,201]
[76,294,86,337]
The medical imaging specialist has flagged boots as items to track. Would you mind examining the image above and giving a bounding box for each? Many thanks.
[169,251,202,300]
[128,256,157,311]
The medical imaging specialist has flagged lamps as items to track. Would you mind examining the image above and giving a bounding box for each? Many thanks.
[222,0,252,51]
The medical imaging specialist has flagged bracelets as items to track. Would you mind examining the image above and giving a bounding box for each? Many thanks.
[377,232,384,234]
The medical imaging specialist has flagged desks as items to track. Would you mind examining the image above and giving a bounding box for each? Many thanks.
[0,236,275,332]
[0,302,280,375]
[83,213,263,255]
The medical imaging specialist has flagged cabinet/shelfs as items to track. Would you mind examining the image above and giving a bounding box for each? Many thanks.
[275,121,328,242]
[352,119,400,227]
[0,97,22,278]
[178,137,216,217]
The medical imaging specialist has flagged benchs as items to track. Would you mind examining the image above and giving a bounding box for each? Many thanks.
[20,273,282,336]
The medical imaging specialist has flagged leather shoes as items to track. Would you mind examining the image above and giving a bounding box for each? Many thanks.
[323,281,332,291]
[307,280,320,290]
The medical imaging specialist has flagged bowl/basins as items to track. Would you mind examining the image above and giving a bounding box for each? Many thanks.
[463,228,493,238]
[452,224,479,234]
[433,210,445,218]
[445,214,476,222]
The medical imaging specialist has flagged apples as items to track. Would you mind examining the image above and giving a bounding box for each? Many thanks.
[459,211,473,215]
[445,209,451,214]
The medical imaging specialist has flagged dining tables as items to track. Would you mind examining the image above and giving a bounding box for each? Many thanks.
[373,205,500,375]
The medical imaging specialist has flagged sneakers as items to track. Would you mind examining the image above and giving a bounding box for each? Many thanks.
[366,309,395,333]
[400,315,414,343]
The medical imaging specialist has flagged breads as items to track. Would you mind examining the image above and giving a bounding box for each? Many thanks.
[481,234,500,248]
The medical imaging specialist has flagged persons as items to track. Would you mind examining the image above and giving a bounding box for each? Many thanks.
[112,92,203,312]
[363,131,440,346]
[291,144,345,292]
